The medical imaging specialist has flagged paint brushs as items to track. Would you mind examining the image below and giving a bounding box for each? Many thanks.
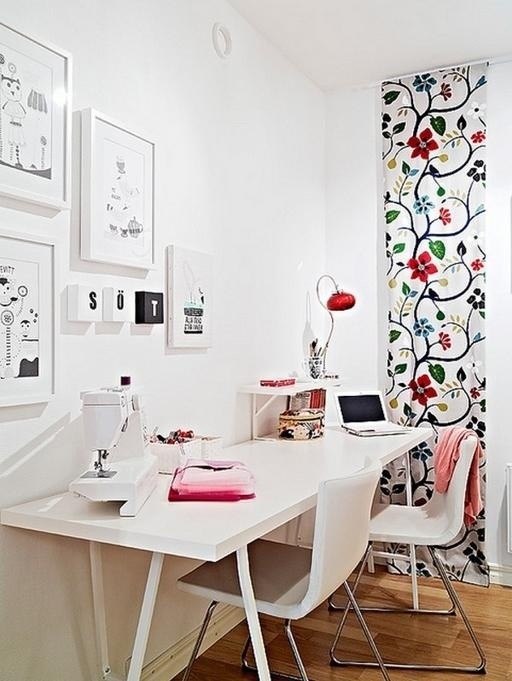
[309,338,322,357]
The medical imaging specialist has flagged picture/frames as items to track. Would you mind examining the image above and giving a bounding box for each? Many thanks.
[0,20,73,411]
[80,107,157,271]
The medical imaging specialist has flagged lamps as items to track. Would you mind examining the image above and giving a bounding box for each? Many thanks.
[315,274,356,379]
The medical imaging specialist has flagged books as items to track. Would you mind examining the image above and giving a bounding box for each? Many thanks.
[287,389,326,414]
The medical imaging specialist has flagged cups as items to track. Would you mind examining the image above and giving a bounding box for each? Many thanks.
[301,356,323,381]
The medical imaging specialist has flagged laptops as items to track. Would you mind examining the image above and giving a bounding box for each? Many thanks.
[333,390,414,437]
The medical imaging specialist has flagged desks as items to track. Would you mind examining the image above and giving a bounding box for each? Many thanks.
[0,423,432,681]
